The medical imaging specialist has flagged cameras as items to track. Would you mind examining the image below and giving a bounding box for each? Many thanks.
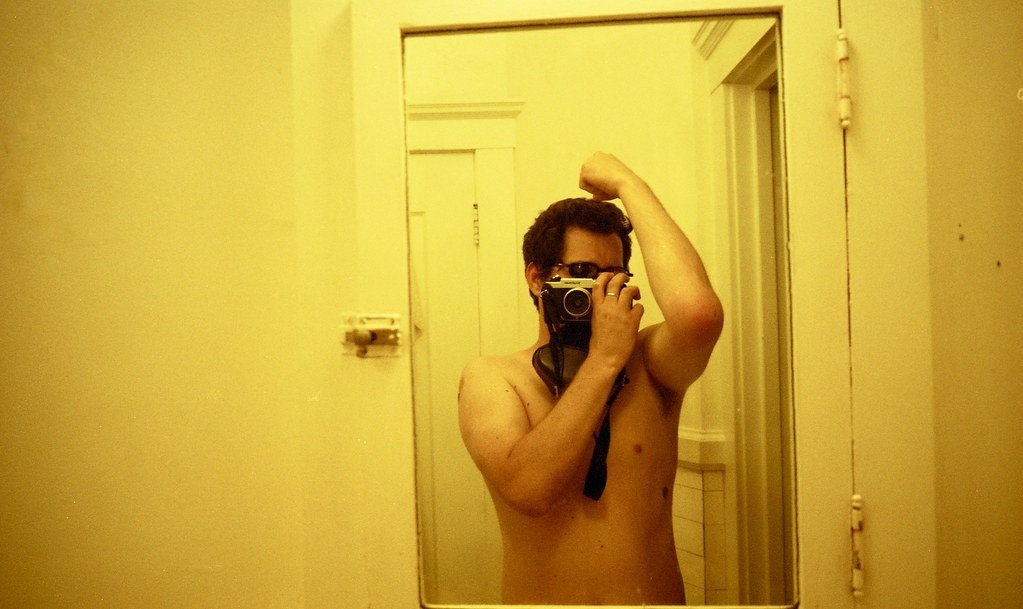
[541,274,595,323]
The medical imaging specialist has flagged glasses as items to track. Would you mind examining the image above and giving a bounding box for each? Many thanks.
[553,261,633,281]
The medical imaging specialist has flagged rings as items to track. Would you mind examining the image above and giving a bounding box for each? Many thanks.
[606,291,619,298]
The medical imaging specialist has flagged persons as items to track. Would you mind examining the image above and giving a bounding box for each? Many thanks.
[456,150,723,606]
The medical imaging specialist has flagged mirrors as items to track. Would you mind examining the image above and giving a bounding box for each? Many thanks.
[400,13,796,606]
